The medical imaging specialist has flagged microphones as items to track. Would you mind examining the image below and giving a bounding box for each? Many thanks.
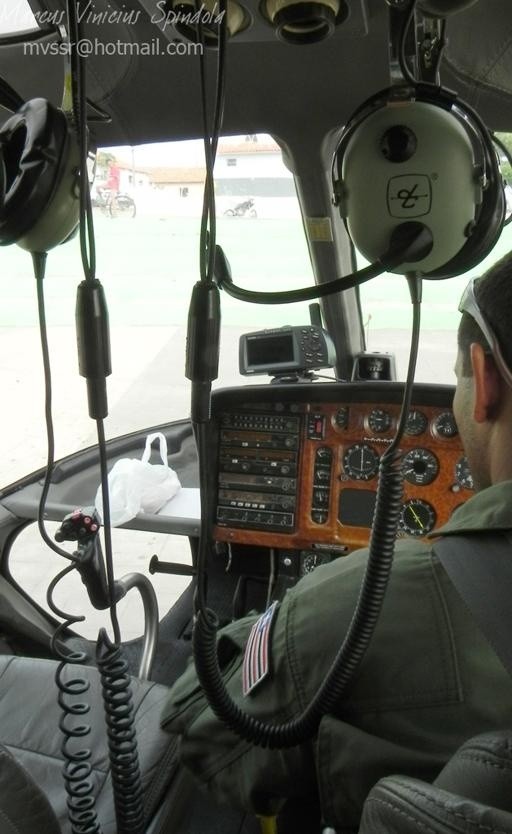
[204,221,434,307]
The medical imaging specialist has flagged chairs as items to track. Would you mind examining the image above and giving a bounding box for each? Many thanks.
[0,649,190,833]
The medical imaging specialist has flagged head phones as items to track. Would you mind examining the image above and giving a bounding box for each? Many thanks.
[0,1,93,252]
[328,0,512,281]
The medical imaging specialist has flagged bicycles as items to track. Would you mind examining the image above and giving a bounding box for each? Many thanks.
[98,189,137,218]
[224,199,257,218]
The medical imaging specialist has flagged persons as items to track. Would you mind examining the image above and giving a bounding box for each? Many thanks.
[96,158,120,215]
[159,251,511,833]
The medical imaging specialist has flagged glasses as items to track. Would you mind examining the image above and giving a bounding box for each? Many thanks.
[457,275,510,383]
